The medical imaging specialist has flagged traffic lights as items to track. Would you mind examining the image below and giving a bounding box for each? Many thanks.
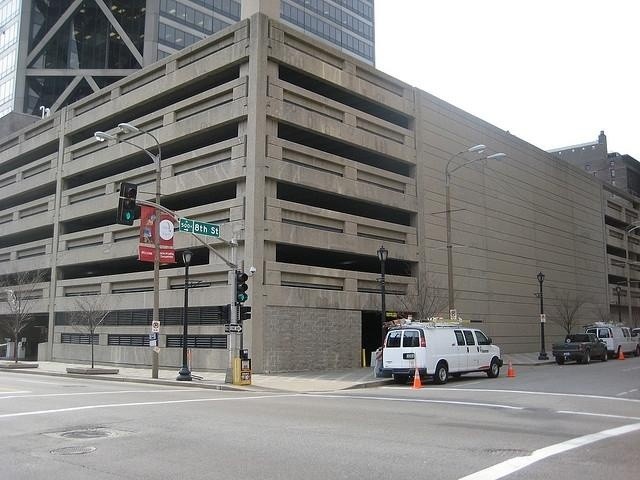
[120,182,138,226]
[235,271,249,303]
[240,306,252,321]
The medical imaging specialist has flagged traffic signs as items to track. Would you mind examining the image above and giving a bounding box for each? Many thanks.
[223,325,244,334]
[177,217,220,238]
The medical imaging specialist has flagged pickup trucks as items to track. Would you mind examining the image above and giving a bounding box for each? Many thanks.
[549,332,610,366]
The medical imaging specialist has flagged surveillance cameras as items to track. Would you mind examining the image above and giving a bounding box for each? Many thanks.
[250,267,256,273]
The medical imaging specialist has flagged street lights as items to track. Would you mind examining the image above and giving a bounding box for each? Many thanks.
[536,271,550,361]
[376,244,390,343]
[93,120,166,378]
[615,285,624,322]
[174,248,195,382]
[443,143,508,318]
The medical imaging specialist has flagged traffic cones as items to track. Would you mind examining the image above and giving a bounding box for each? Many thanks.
[618,346,626,360]
[505,360,517,378]
[410,367,424,390]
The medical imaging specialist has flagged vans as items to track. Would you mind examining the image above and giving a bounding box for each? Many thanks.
[378,313,502,384]
[584,321,640,358]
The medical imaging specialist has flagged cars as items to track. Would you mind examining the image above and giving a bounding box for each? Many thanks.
[632,328,640,335]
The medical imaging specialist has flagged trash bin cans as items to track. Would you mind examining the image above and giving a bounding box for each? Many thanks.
[233,358,251,387]
